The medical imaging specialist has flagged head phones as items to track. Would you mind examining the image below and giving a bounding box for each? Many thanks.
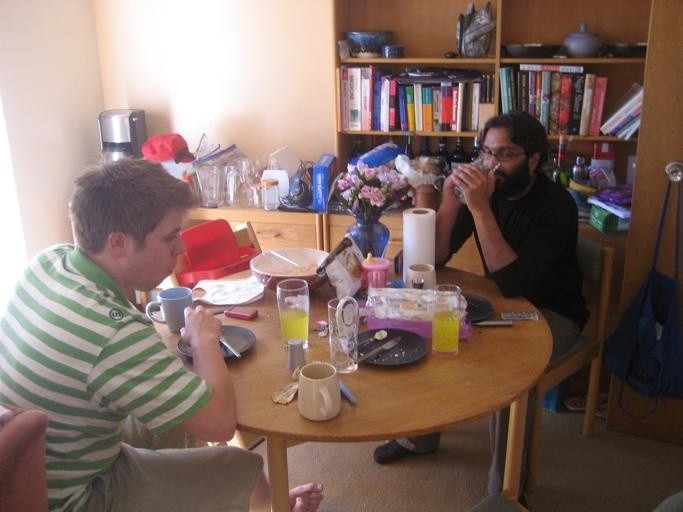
[288,165,312,201]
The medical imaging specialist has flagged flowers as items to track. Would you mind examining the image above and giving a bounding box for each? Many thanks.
[334,164,409,220]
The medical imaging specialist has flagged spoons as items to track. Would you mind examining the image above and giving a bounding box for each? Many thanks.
[356,330,387,346]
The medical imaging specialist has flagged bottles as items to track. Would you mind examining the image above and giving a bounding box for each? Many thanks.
[562,22,603,58]
[353,133,483,179]
[286,339,306,370]
[551,145,587,192]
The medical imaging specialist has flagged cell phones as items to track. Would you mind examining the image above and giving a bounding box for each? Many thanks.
[223,306,259,320]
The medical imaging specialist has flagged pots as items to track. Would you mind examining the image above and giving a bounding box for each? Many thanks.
[251,237,352,292]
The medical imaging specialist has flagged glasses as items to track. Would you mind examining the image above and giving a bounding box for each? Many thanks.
[477,147,533,161]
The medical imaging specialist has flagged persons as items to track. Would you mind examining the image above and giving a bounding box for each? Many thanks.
[0,159,323,511]
[374,113,583,508]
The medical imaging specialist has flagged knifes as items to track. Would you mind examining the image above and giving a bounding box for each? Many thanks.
[217,335,241,359]
[354,335,401,366]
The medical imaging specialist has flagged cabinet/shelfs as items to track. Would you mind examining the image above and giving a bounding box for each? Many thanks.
[495,0,681,452]
[176,207,323,253]
[319,211,488,279]
[332,0,500,214]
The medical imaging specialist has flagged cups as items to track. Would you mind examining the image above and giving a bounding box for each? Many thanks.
[145,287,193,334]
[276,279,309,349]
[194,155,282,212]
[297,361,341,422]
[328,297,359,373]
[361,257,391,291]
[432,284,462,357]
[455,153,501,205]
[407,265,437,290]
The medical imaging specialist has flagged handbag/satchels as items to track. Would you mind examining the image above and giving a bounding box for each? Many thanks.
[601,266,681,399]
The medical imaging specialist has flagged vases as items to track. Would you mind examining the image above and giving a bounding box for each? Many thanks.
[349,220,389,259]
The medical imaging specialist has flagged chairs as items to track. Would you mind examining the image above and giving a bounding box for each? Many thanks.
[525,237,613,490]
[168,219,267,289]
[456,487,682,512]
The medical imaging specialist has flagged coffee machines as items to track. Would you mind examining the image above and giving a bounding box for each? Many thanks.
[97,109,147,164]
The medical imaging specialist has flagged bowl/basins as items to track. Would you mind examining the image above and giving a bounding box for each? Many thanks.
[606,42,646,59]
[343,31,394,58]
[504,42,561,57]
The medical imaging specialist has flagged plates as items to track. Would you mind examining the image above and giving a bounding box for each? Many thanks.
[355,328,427,368]
[457,292,494,322]
[176,325,255,361]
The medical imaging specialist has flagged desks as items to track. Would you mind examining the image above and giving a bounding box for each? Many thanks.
[147,258,553,512]
